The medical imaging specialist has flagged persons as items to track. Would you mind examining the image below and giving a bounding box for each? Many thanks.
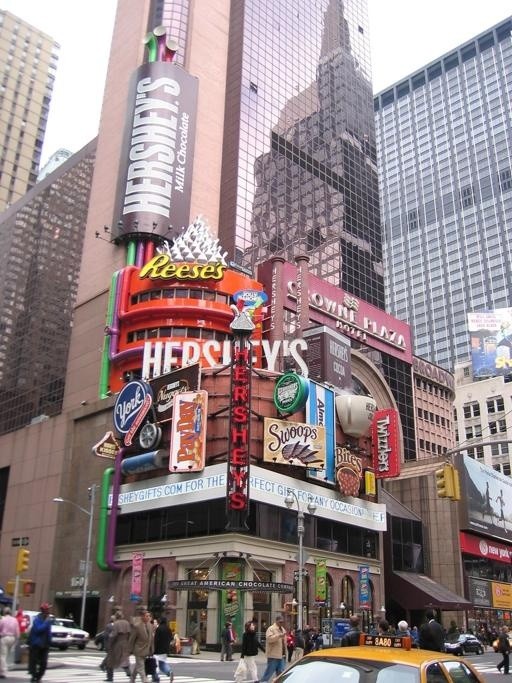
[99,610,181,683]
[339,608,512,674]
[219,615,322,682]
[188,620,202,655]
[0,600,51,683]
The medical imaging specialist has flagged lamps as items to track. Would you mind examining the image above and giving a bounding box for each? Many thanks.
[94,221,186,245]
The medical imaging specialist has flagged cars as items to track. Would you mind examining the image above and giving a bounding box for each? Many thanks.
[493,630,512,652]
[15,610,104,650]
[444,634,484,655]
[272,634,485,683]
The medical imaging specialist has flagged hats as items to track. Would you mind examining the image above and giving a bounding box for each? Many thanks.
[276,616,283,623]
[39,603,52,610]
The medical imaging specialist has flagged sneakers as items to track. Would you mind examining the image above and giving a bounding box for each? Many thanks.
[497,665,512,675]
[169,671,173,682]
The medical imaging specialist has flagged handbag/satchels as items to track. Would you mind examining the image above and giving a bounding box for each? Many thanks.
[145,657,156,674]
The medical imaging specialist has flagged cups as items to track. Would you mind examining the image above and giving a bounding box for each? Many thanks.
[335,394,376,439]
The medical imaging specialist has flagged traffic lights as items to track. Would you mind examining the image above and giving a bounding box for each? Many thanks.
[436,463,460,501]
[15,548,30,573]
[6,579,35,598]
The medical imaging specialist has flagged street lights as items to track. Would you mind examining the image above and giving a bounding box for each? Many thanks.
[285,491,315,647]
[53,484,98,629]
